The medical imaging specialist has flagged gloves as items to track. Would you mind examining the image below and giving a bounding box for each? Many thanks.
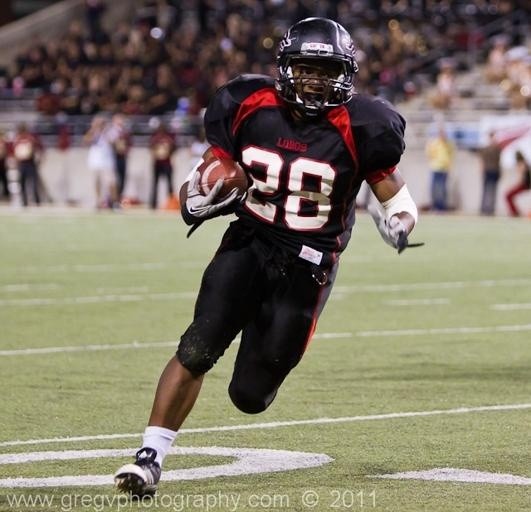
[364,201,409,255]
[185,173,247,220]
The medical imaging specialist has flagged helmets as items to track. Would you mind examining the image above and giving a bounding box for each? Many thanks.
[275,17,360,120]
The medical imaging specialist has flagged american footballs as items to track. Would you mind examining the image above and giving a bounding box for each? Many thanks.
[196,155,248,201]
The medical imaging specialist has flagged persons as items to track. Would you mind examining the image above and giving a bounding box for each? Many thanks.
[113,16,417,500]
[1,1,531,216]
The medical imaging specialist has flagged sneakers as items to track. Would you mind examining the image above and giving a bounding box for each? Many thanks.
[112,449,165,498]
